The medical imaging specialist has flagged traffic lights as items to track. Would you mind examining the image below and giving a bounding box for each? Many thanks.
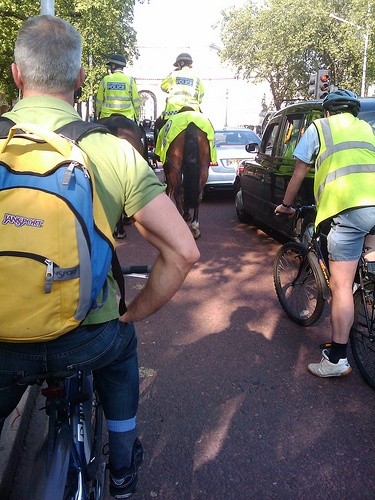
[317,70,329,100]
[308,72,318,98]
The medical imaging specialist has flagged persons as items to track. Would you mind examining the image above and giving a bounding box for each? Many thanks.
[0,105,8,115]
[96,55,156,170]
[0,16,200,499]
[154,53,204,162]
[274,90,375,377]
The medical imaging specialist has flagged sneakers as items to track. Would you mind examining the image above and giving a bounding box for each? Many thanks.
[307,349,353,377]
[103,431,143,499]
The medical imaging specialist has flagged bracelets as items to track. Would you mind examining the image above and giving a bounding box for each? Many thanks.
[282,201,292,208]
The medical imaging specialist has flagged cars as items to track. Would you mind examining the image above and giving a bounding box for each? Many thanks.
[233,96,375,272]
[138,119,156,157]
[203,127,272,202]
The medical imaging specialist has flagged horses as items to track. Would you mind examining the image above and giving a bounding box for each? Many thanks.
[94,105,214,240]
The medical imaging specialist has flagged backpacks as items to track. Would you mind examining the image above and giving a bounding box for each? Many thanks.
[0,116,114,342]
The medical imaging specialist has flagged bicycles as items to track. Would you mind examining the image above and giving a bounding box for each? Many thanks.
[272,204,375,390]
[27,263,153,500]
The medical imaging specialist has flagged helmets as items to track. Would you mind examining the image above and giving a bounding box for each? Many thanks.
[106,55,126,67]
[173,53,193,67]
[321,89,361,109]
